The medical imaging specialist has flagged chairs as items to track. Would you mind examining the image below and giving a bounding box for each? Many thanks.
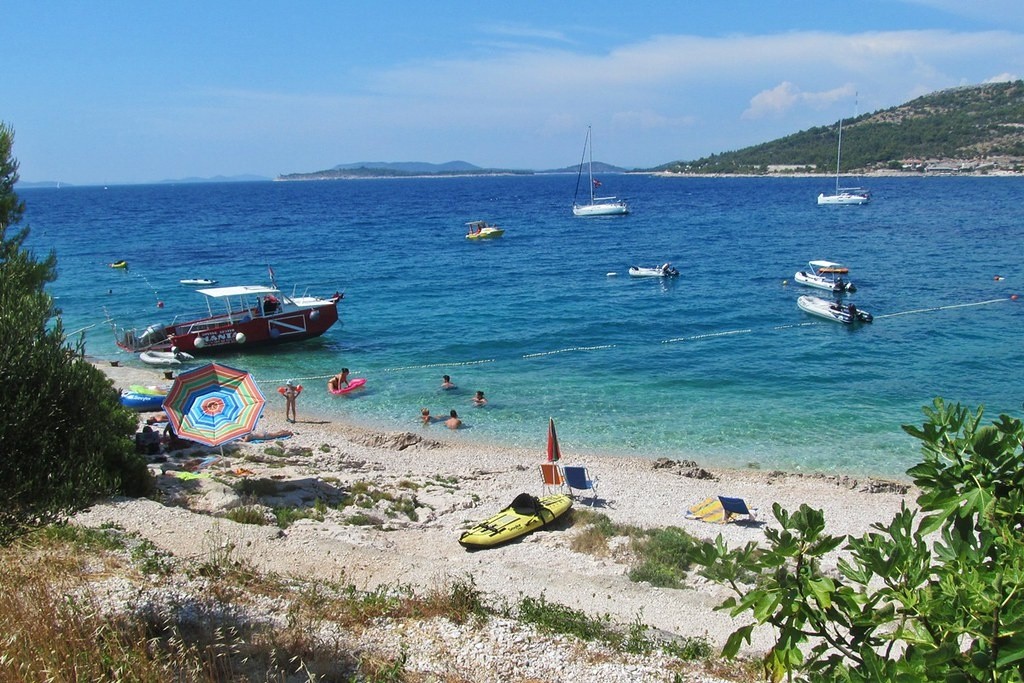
[538,463,570,499]
[718,493,758,529]
[561,466,599,508]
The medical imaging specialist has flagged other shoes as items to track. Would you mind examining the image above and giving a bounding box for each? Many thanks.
[291,420,296,423]
[286,418,291,422]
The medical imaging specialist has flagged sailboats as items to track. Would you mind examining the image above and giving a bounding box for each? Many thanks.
[573,125,632,216]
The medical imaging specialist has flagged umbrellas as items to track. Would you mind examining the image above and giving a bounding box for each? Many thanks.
[161,363,267,448]
[547,419,561,495]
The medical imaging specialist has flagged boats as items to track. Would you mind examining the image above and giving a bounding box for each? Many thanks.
[816,118,871,206]
[628,263,679,278]
[116,257,343,353]
[180,279,217,284]
[796,295,872,322]
[121,384,164,412]
[794,260,857,293]
[465,221,505,239]
[140,351,195,366]
[332,378,367,395]
[111,260,128,268]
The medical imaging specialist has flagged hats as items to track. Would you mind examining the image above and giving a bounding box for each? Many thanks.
[286,379,292,385]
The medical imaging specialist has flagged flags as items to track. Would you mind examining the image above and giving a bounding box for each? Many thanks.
[593,178,602,188]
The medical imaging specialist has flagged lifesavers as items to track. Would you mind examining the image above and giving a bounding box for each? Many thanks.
[278,384,303,393]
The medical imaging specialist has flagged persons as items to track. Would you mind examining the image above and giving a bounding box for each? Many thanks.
[662,262,671,272]
[472,391,487,403]
[328,368,350,390]
[421,408,430,420]
[281,379,301,423]
[143,413,294,468]
[441,375,453,388]
[445,411,461,428]
[263,294,279,312]
[476,223,498,233]
